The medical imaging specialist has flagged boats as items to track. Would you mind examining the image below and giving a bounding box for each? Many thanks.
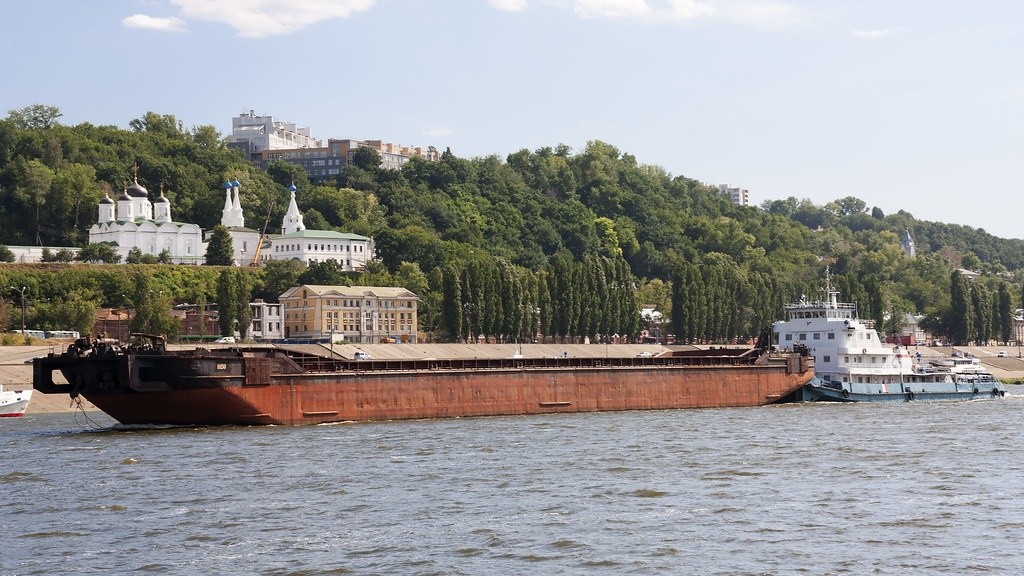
[0,384,33,418]
[764,265,1008,404]
[29,295,818,433]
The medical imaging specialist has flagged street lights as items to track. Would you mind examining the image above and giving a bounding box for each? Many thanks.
[10,286,26,335]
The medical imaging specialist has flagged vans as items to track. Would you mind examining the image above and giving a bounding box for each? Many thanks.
[354,351,369,360]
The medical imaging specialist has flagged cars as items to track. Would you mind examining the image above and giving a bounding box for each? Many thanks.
[998,351,1007,357]
[380,335,404,344]
[214,337,237,344]
[636,352,652,359]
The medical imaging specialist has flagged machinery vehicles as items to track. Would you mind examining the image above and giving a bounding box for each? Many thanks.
[249,200,275,267]
